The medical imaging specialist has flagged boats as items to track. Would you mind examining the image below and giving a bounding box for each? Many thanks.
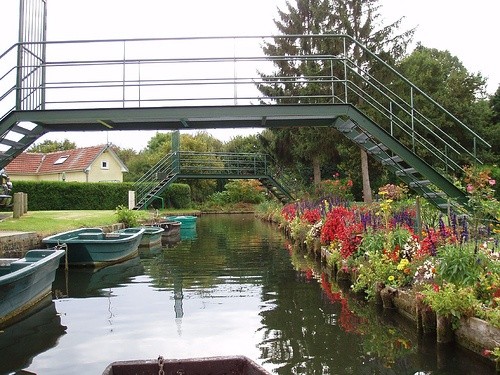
[98,352,274,375]
[43,223,145,273]
[0,246,66,328]
[156,218,183,229]
[166,215,198,222]
[121,226,165,248]
